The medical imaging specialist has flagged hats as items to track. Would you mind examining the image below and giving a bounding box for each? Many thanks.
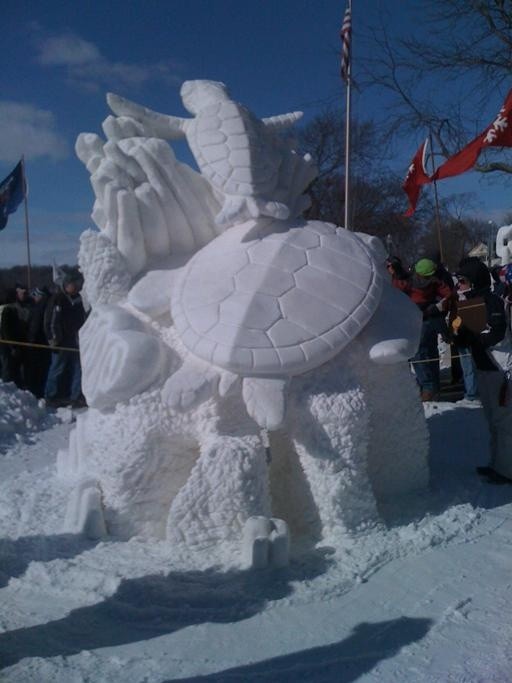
[415,258,436,276]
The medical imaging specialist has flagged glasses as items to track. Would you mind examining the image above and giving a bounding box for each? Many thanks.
[458,278,470,285]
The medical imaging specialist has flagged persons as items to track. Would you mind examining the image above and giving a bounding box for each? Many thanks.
[386,251,511,487]
[1,275,87,410]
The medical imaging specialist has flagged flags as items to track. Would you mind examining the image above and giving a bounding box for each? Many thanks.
[0,157,27,230]
[431,88,512,179]
[401,136,431,217]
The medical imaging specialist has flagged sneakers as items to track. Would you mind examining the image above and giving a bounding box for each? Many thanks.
[476,466,511,485]
[421,391,441,402]
